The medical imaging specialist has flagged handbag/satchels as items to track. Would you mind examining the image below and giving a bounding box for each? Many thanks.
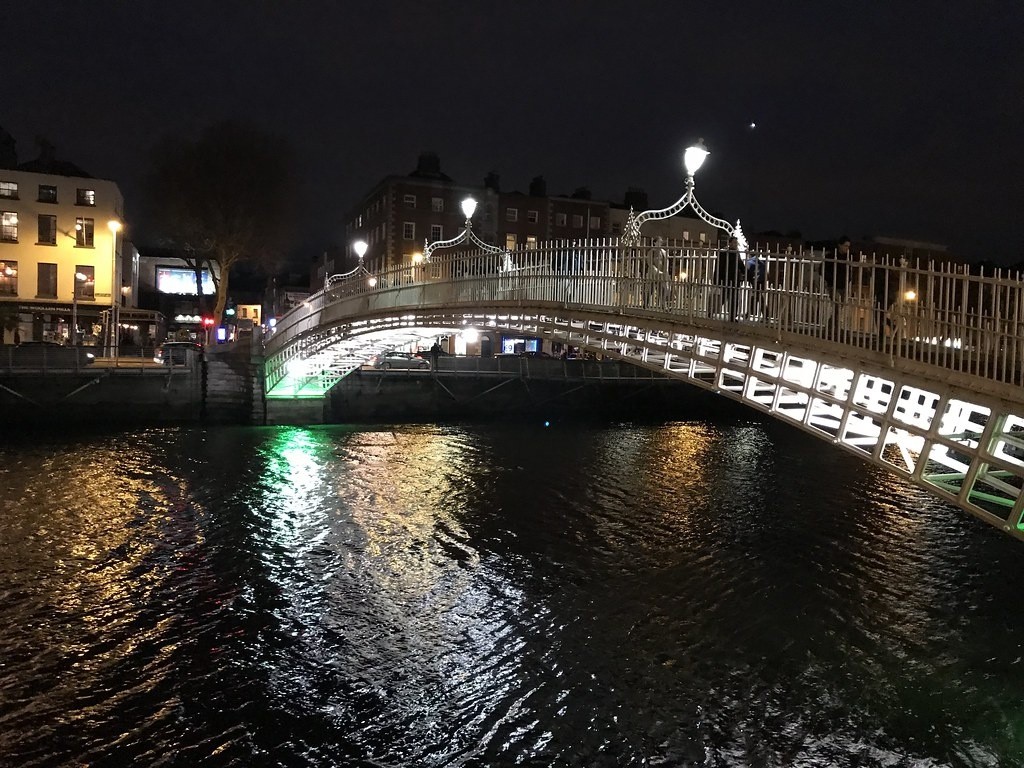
[708,286,720,314]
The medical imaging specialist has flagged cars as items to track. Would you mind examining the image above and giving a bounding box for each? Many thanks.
[11,341,94,368]
[373,352,430,370]
[517,351,558,360]
[415,351,452,359]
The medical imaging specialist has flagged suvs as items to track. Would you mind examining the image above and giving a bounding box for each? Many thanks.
[153,341,203,365]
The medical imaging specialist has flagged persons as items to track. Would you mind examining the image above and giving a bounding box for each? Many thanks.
[818,239,851,336]
[741,247,769,323]
[431,343,440,357]
[644,235,669,307]
[707,237,746,322]
[484,258,498,274]
[862,251,898,351]
[451,252,467,277]
[14,327,20,345]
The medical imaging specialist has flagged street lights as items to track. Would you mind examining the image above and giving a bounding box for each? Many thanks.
[225,308,236,342]
[71,271,87,345]
[906,289,916,314]
[106,218,123,359]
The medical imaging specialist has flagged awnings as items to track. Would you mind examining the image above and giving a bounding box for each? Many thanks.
[0,295,114,307]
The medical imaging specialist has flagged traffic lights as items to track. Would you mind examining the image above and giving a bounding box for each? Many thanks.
[205,318,214,330]
